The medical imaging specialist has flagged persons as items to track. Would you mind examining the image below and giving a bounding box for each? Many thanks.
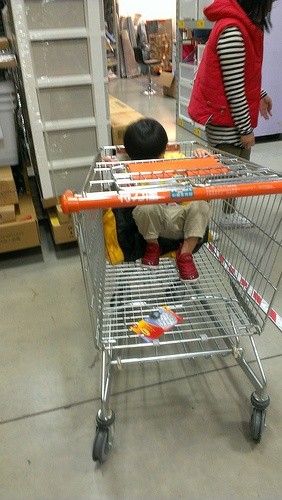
[103,118,213,284]
[187,0,275,230]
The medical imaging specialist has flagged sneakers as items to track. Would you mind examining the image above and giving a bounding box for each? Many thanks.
[209,230,219,241]
[220,210,254,229]
[141,242,161,270]
[176,243,199,283]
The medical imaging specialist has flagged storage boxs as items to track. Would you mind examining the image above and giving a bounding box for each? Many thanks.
[0,195,41,254]
[160,70,175,97]
[0,205,16,223]
[0,169,20,205]
[48,204,78,244]
[108,93,145,150]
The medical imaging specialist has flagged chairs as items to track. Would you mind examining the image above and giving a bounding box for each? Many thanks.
[133,47,162,96]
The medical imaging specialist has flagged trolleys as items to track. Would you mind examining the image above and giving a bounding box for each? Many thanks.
[59,138,282,465]
